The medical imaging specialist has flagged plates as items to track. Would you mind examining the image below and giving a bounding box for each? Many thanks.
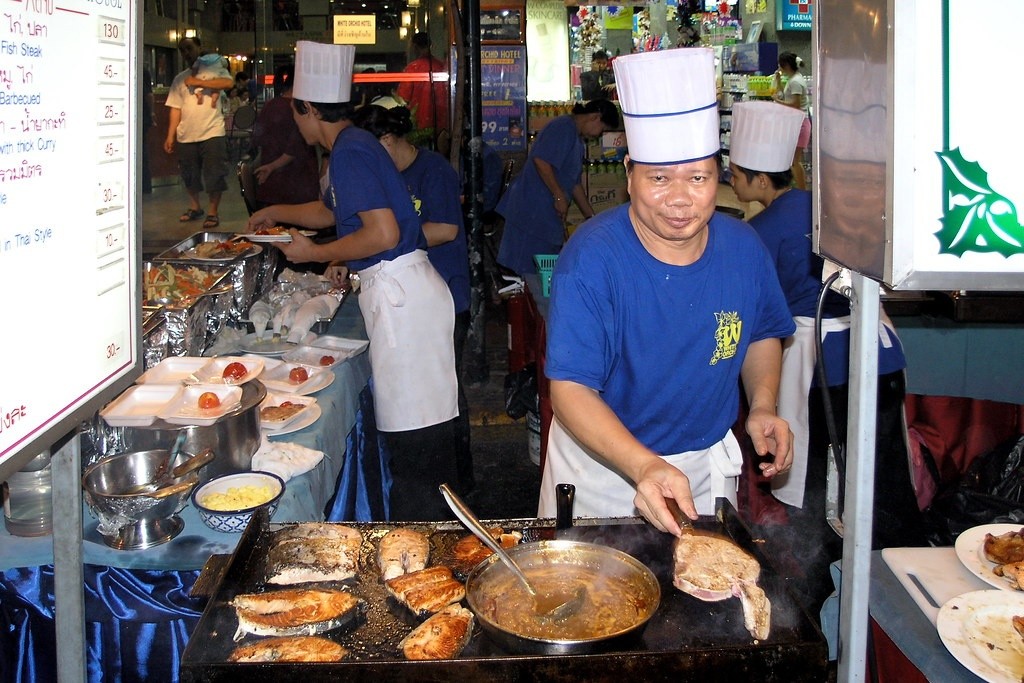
[954,523,1024,594]
[302,371,335,395]
[238,328,318,357]
[233,231,317,242]
[262,404,322,435]
[937,589,1024,682]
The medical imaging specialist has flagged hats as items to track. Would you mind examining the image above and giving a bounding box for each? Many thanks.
[612,46,720,165]
[293,41,355,104]
[729,101,805,173]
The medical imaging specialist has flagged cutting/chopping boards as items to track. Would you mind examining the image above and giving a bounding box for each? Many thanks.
[881,547,997,630]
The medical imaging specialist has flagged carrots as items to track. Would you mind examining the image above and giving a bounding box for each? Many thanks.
[147,266,216,297]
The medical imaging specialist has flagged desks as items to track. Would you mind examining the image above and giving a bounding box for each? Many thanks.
[506,277,1024,527]
[0,292,388,683]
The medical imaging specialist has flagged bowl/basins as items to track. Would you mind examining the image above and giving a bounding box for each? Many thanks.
[96,332,368,424]
[191,470,286,531]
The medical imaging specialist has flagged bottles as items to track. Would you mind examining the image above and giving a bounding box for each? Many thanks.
[527,131,536,144]
[749,75,772,90]
[587,158,626,177]
[3,451,53,537]
[531,99,575,118]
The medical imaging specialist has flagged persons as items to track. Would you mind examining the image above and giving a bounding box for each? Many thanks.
[244,38,464,522]
[141,31,473,414]
[727,100,936,548]
[538,46,797,538]
[493,99,619,335]
[578,51,621,98]
[771,51,810,194]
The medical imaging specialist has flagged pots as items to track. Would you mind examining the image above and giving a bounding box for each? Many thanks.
[466,483,662,658]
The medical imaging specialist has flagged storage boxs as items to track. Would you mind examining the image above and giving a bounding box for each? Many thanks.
[528,116,551,131]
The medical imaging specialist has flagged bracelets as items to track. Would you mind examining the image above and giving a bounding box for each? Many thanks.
[553,194,567,202]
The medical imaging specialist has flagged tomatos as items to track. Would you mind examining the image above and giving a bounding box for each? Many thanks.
[198,391,221,409]
[320,355,335,365]
[222,362,247,378]
[289,367,308,381]
[254,230,269,235]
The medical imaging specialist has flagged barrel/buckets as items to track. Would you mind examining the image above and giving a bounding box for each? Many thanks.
[125,378,268,483]
[527,410,540,467]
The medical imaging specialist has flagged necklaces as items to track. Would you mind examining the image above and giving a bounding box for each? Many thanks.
[401,144,415,169]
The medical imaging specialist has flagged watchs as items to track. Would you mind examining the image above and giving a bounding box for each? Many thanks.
[583,212,597,221]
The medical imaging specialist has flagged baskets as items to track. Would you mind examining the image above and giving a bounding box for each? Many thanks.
[535,255,560,298]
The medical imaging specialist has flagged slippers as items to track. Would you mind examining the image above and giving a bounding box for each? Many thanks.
[179,208,203,222]
[202,213,219,229]
